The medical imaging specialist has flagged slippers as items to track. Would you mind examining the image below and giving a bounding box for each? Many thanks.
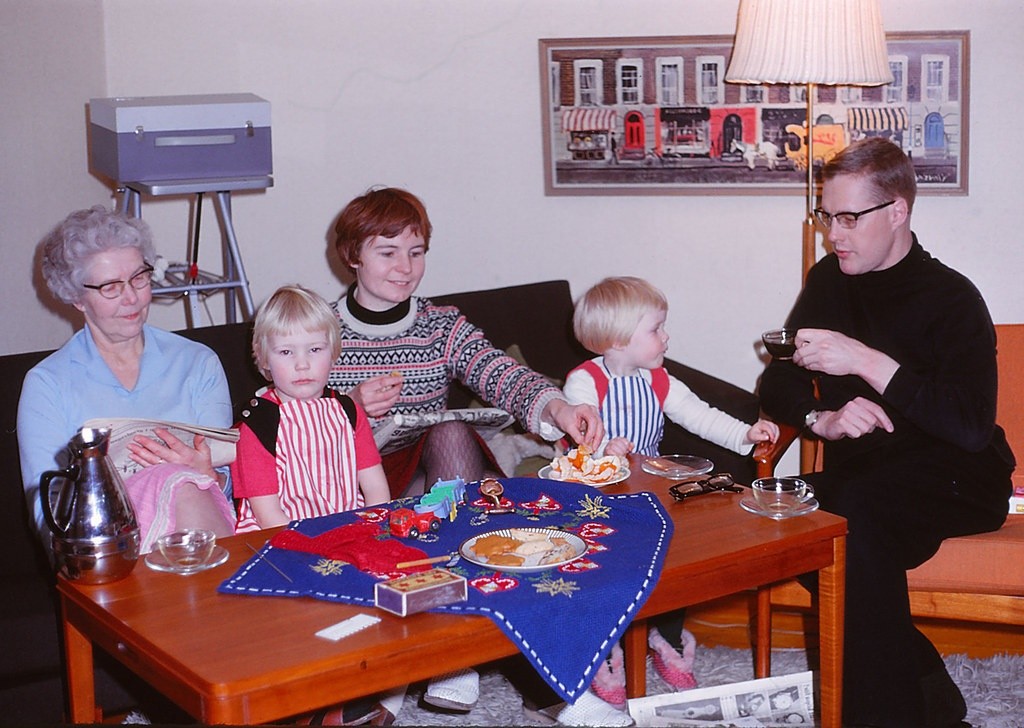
[423,667,480,711]
[522,690,635,727]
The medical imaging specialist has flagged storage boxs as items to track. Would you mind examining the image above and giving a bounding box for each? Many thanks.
[374,568,468,618]
[89,92,273,182]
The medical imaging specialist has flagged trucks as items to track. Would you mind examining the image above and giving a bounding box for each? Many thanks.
[390,508,442,540]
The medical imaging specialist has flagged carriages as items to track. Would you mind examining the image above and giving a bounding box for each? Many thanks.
[728,125,846,174]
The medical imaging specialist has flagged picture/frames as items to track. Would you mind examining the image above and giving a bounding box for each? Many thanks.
[537,29,970,198]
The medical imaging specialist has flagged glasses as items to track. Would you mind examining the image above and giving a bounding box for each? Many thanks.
[669,473,743,502]
[813,200,896,230]
[83,261,155,300]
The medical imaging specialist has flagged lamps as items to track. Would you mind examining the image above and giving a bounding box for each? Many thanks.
[725,1,895,291]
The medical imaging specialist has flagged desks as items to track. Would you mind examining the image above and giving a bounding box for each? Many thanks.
[112,174,275,329]
[57,454,846,728]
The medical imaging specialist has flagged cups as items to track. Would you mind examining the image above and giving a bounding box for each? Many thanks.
[149,527,217,568]
[751,476,814,517]
[761,327,797,360]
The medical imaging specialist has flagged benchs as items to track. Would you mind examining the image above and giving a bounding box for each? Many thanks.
[684,323,1023,660]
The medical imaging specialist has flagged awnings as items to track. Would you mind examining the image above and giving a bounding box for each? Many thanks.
[848,107,909,134]
[562,108,617,132]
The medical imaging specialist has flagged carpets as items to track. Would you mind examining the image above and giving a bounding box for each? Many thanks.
[123,645,1024,728]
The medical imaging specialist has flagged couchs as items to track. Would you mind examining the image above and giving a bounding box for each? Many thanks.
[0,280,762,728]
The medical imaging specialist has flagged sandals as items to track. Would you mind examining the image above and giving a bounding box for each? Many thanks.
[291,687,396,728]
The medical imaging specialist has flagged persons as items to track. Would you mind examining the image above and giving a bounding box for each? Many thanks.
[564,276,779,708]
[15,207,235,554]
[754,137,1017,728]
[326,183,607,708]
[233,287,394,728]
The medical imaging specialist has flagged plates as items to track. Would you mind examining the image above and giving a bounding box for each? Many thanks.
[641,454,715,480]
[144,543,229,575]
[739,495,819,521]
[459,527,588,574]
[537,463,632,488]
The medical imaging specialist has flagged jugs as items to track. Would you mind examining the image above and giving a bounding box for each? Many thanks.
[38,427,142,585]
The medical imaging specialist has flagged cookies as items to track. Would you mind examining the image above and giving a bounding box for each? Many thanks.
[469,528,579,565]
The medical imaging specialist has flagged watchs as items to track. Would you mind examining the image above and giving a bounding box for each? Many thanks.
[803,409,822,430]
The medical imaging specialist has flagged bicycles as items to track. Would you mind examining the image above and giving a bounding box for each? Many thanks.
[640,147,682,172]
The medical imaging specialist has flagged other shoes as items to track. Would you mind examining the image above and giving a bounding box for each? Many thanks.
[593,642,627,712]
[649,627,700,690]
